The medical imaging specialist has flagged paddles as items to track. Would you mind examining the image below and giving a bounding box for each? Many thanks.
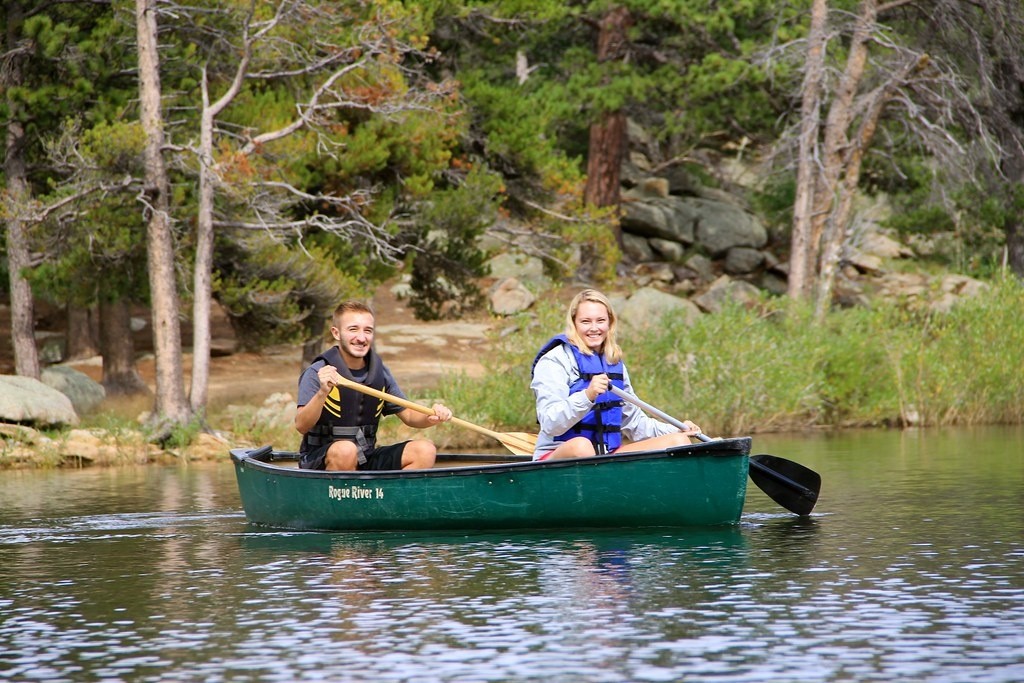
[608,385,822,516]
[330,373,538,456]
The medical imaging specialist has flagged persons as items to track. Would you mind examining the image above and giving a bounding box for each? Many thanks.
[294,301,452,472]
[529,288,702,462]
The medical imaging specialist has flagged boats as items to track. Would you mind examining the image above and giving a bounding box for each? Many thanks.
[224,436,757,531]
